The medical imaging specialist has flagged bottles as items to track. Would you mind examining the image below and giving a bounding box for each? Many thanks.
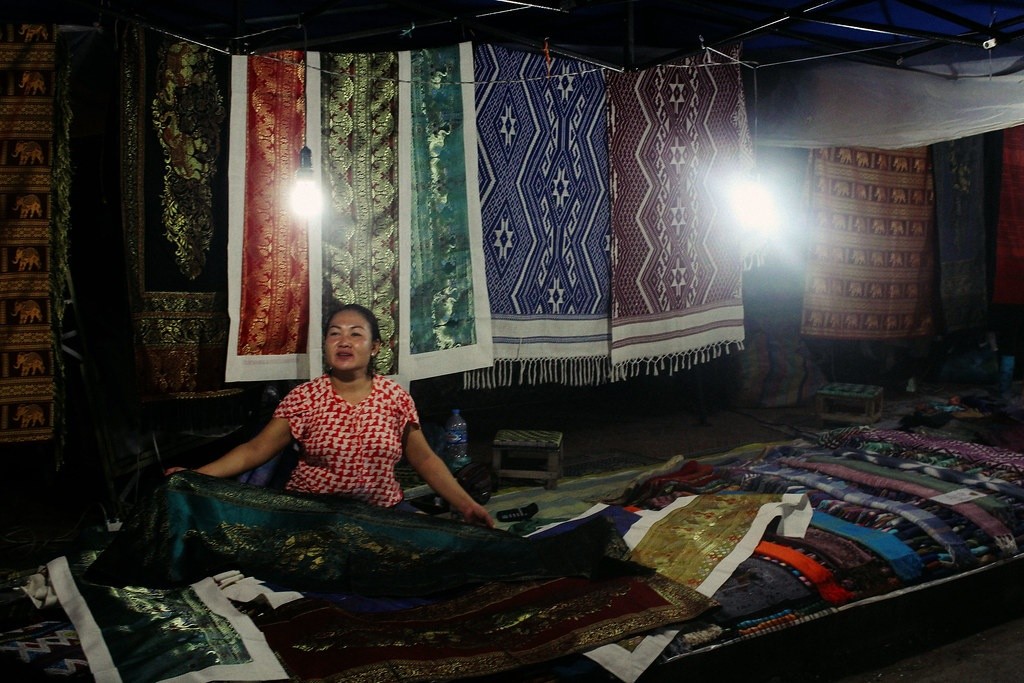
[1000,356,1014,393]
[444,409,467,463]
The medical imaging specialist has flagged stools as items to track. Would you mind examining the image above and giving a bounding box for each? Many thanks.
[814,381,883,431]
[491,429,563,493]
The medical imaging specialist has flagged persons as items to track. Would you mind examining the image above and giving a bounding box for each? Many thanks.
[164,303,496,529]
